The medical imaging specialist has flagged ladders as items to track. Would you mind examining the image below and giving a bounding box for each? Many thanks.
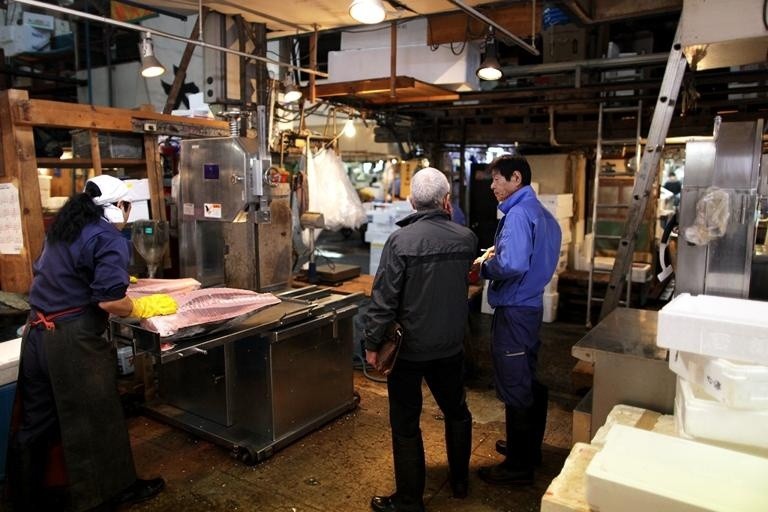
[599,17,682,321]
[585,53,644,330]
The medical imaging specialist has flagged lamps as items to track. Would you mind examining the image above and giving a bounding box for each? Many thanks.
[475,26,505,82]
[348,0,387,26]
[140,31,166,79]
[283,75,302,102]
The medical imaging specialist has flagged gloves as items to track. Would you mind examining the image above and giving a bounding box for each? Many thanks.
[121,294,178,318]
[129,275,138,283]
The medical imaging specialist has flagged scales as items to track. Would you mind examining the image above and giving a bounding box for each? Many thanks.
[300,211,362,283]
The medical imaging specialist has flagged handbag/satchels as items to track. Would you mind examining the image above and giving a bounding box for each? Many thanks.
[374,324,402,375]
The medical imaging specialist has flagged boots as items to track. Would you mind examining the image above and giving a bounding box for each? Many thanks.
[483,404,535,485]
[103,474,165,504]
[445,413,473,498]
[7,441,66,510]
[496,384,549,463]
[371,429,426,512]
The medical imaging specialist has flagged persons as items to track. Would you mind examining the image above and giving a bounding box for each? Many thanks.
[14,174,167,508]
[473,154,563,489]
[358,166,475,512]
